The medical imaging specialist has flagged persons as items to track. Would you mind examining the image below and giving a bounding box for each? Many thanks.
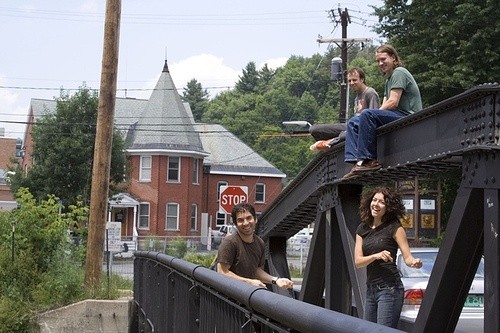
[307,67,379,150]
[342,44,422,179]
[354,187,422,328]
[217,205,295,291]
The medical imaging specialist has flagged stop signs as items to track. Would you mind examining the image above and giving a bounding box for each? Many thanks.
[219,185,248,214]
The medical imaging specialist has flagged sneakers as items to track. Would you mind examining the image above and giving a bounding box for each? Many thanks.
[341,163,372,179]
[352,160,382,174]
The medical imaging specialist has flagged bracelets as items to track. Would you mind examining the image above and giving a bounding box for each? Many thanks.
[272,275,278,284]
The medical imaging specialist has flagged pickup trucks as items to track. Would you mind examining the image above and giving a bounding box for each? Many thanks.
[210,225,237,250]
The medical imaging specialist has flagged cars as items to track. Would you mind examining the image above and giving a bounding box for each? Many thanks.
[392,246,485,332]
